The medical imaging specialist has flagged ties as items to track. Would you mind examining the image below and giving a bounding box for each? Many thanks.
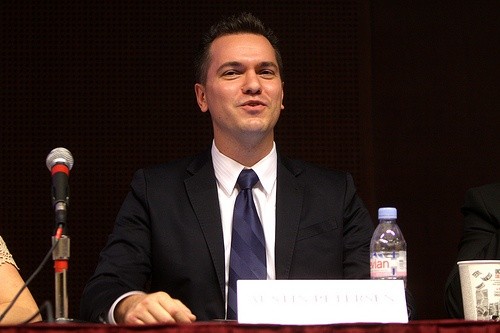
[226,169,266,321]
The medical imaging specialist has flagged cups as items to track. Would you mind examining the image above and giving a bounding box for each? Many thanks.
[457,260,500,319]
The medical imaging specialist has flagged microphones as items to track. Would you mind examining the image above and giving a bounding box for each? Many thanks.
[46,148,74,227]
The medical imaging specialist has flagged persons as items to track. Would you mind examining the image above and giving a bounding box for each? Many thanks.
[81,13,413,326]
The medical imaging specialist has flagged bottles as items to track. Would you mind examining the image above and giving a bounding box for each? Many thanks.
[370,208,407,280]
